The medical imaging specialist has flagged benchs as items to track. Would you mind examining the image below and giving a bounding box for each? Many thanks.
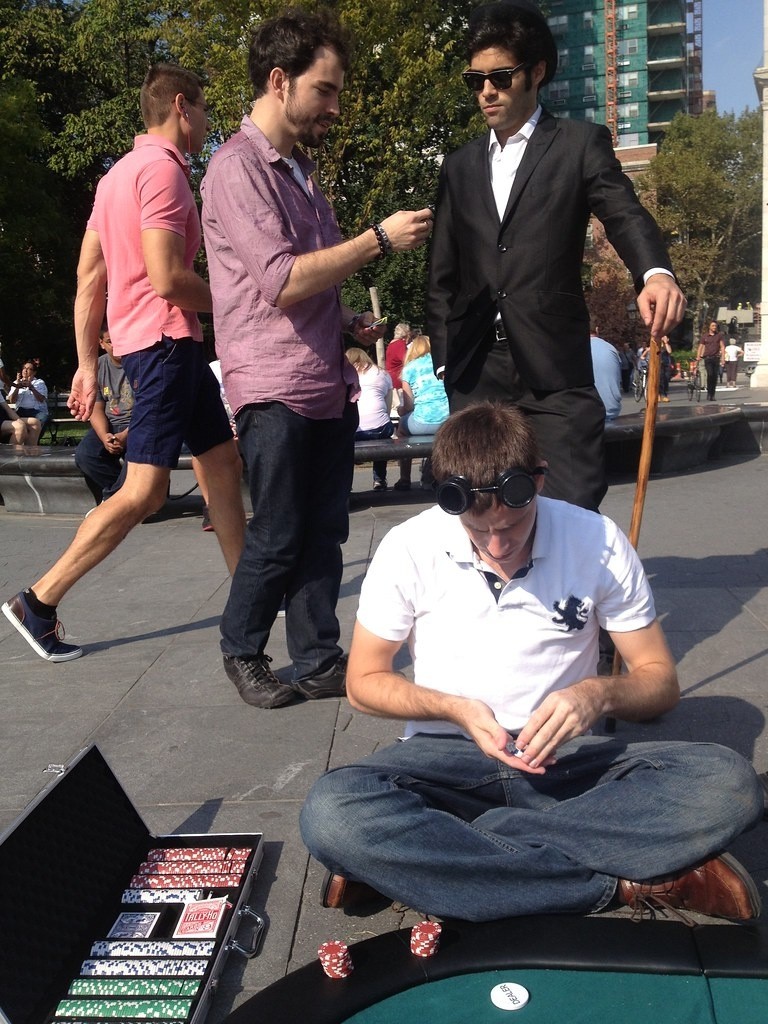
[0,402,768,515]
[8,386,90,445]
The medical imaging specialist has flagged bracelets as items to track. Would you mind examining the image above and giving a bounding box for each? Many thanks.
[348,314,360,327]
[377,224,391,249]
[372,226,385,259]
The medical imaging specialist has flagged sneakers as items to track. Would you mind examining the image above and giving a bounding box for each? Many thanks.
[294,655,352,699]
[202,504,213,531]
[1,592,83,662]
[224,652,295,708]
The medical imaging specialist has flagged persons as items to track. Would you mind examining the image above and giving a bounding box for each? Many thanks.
[637,335,672,402]
[725,338,745,388]
[346,324,451,489]
[1,61,286,662]
[719,367,724,383]
[301,399,762,922]
[200,14,432,709]
[697,321,725,401]
[621,342,636,394]
[0,343,49,447]
[590,321,621,419]
[74,321,134,521]
[427,0,688,677]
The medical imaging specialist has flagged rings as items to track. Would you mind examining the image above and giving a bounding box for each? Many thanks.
[424,221,429,230]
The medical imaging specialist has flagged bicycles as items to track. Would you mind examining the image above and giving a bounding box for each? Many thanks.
[685,355,702,402]
[631,360,647,402]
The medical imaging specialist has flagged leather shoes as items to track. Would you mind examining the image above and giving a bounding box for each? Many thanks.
[320,868,371,910]
[618,849,763,928]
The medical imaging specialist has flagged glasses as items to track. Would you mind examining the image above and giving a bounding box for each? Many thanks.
[461,61,527,91]
[436,467,537,516]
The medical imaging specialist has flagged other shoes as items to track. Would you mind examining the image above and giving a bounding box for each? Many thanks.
[421,477,435,492]
[395,480,412,491]
[707,395,716,401]
[373,480,387,489]
[663,397,669,402]
[657,395,661,403]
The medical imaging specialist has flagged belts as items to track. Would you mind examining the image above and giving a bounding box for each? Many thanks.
[484,322,507,342]
